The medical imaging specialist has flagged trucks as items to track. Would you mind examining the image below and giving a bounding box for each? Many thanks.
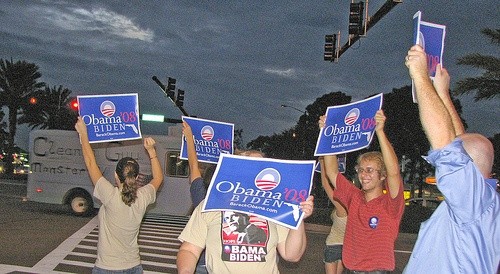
[26,129,214,217]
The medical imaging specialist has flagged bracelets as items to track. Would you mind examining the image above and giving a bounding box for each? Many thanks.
[148,154,158,160]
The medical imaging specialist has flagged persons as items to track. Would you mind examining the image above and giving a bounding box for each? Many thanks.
[314,108,406,274]
[176,142,315,274]
[74,113,165,274]
[401,43,500,274]
[317,114,350,274]
[180,117,229,274]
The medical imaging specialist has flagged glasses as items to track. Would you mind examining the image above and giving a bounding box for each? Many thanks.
[355,167,380,174]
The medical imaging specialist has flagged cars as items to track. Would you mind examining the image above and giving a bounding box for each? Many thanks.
[404,196,441,210]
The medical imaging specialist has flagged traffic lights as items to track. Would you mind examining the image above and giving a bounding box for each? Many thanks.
[72,102,78,108]
[30,97,37,104]
